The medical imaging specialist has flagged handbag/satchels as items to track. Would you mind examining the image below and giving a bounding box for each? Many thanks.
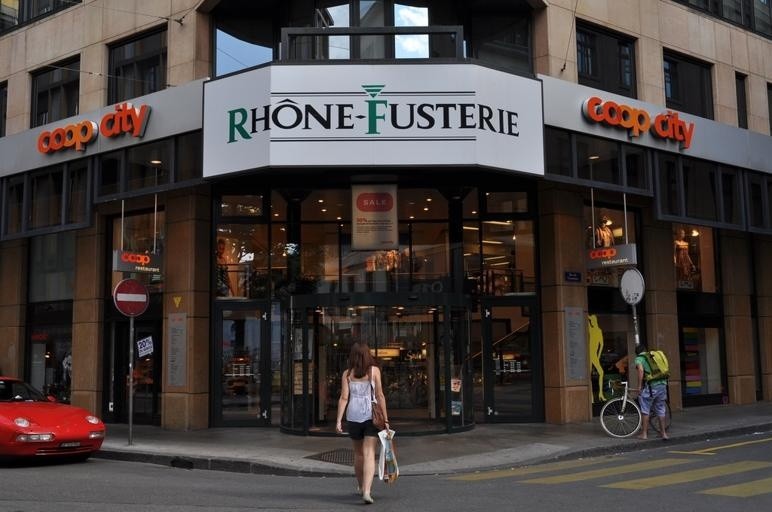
[376,429,399,484]
[371,401,386,430]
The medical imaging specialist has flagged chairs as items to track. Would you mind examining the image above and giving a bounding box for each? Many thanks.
[0,384,11,400]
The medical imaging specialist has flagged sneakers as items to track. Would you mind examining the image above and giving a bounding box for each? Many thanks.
[357,486,374,503]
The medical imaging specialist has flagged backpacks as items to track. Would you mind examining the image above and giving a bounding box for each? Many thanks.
[639,349,670,383]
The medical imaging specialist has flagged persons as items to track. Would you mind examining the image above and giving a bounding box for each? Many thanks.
[674,229,696,288]
[335,342,390,503]
[634,345,670,440]
[587,314,607,404]
[595,215,618,288]
[216,239,235,297]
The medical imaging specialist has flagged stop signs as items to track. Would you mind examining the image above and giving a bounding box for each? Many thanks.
[114,278,151,318]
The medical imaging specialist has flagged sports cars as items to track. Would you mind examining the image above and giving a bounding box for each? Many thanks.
[0,377,106,462]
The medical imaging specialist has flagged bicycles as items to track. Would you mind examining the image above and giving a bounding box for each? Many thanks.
[600,382,673,437]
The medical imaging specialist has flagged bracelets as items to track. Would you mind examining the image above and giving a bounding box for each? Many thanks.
[384,421,389,424]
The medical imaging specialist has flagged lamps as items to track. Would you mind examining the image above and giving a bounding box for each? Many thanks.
[463,220,512,266]
